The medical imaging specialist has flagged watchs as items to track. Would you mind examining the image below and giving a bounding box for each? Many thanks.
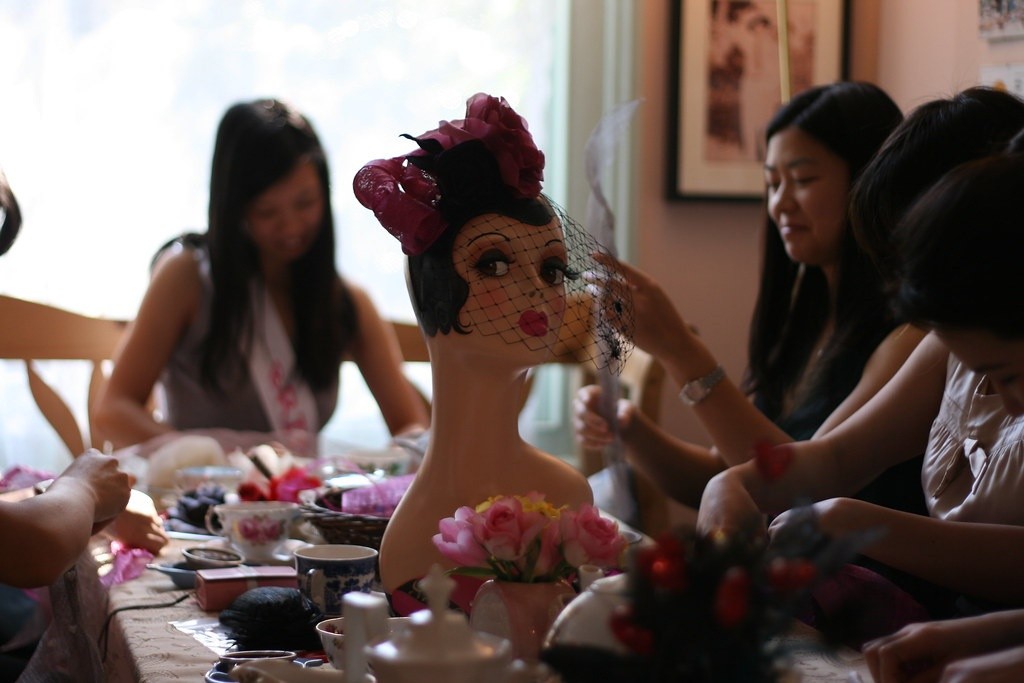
[678,363,727,408]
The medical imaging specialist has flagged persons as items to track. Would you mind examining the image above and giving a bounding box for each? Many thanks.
[688,85,1023,620]
[859,129,1024,683]
[707,0,813,165]
[93,98,430,465]
[350,87,603,617]
[0,447,133,683]
[572,77,906,514]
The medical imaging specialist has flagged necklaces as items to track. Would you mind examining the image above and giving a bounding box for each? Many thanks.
[815,334,839,359]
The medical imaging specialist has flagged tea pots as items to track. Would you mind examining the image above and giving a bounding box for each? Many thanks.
[540,562,634,653]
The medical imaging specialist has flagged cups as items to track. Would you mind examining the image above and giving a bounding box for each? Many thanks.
[140,462,514,683]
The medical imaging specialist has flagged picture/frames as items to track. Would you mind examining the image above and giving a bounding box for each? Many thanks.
[673,0,851,204]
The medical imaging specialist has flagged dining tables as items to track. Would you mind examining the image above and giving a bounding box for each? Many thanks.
[75,432,903,682]
